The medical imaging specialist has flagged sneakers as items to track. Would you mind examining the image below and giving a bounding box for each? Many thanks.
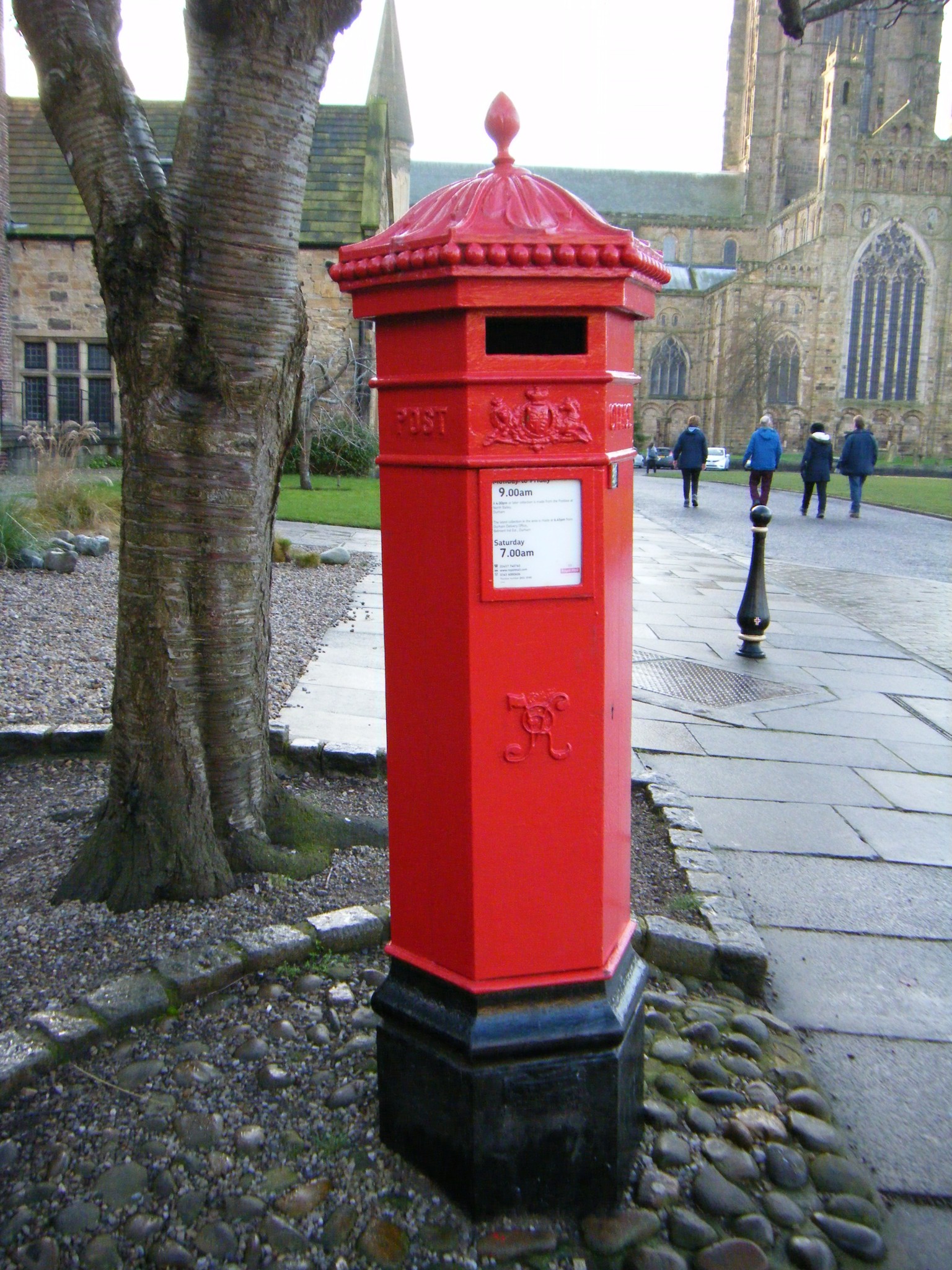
[816,509,825,518]
[750,500,761,510]
[801,507,808,516]
[684,500,689,507]
[849,512,859,518]
[692,494,698,506]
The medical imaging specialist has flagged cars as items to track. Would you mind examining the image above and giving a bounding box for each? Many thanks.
[743,455,752,471]
[654,446,680,470]
[632,446,645,468]
[705,447,730,471]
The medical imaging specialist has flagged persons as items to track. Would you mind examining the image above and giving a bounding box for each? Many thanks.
[672,415,708,507]
[798,422,834,518]
[742,415,782,512]
[645,442,659,474]
[833,415,877,519]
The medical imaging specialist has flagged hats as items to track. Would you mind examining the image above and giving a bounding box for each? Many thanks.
[811,423,824,434]
